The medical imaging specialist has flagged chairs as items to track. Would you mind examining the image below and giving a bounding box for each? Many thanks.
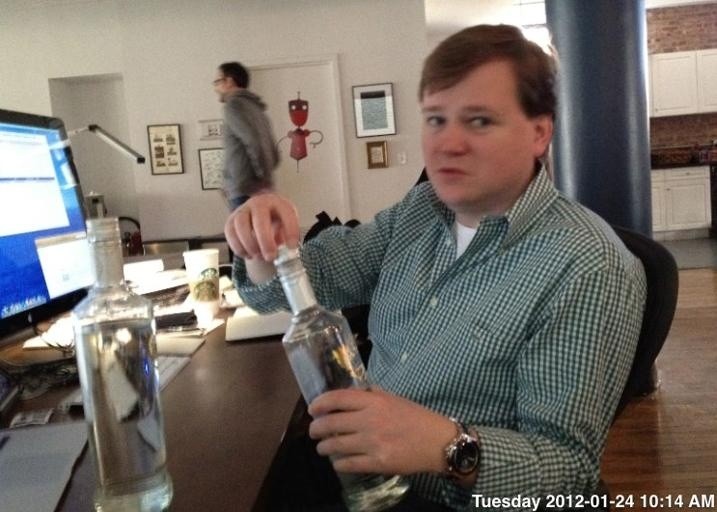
[342,223,678,510]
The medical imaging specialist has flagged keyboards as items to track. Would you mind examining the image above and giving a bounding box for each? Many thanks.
[57,356,190,414]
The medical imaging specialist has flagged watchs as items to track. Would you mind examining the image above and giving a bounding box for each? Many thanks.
[443,417,481,482]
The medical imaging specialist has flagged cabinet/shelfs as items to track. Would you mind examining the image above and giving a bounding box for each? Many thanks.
[651,165,712,241]
[648,48,717,118]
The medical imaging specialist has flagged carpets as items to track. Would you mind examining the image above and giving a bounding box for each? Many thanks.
[658,238,717,269]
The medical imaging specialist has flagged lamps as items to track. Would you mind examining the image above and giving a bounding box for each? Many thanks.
[67,123,145,163]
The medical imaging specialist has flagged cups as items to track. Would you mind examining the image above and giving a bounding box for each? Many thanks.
[182,248,220,310]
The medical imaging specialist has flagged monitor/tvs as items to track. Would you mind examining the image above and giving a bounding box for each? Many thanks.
[0,108,96,376]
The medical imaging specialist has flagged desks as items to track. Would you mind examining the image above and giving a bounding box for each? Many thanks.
[0,286,301,511]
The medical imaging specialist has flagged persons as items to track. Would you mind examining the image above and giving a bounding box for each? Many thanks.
[214,62,281,264]
[223,24,647,511]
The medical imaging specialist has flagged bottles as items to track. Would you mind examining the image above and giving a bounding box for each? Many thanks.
[69,219,177,512]
[270,239,409,510]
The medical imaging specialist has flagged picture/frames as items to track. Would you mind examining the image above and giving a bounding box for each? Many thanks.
[352,82,397,138]
[198,147,227,190]
[367,140,388,169]
[147,124,184,175]
